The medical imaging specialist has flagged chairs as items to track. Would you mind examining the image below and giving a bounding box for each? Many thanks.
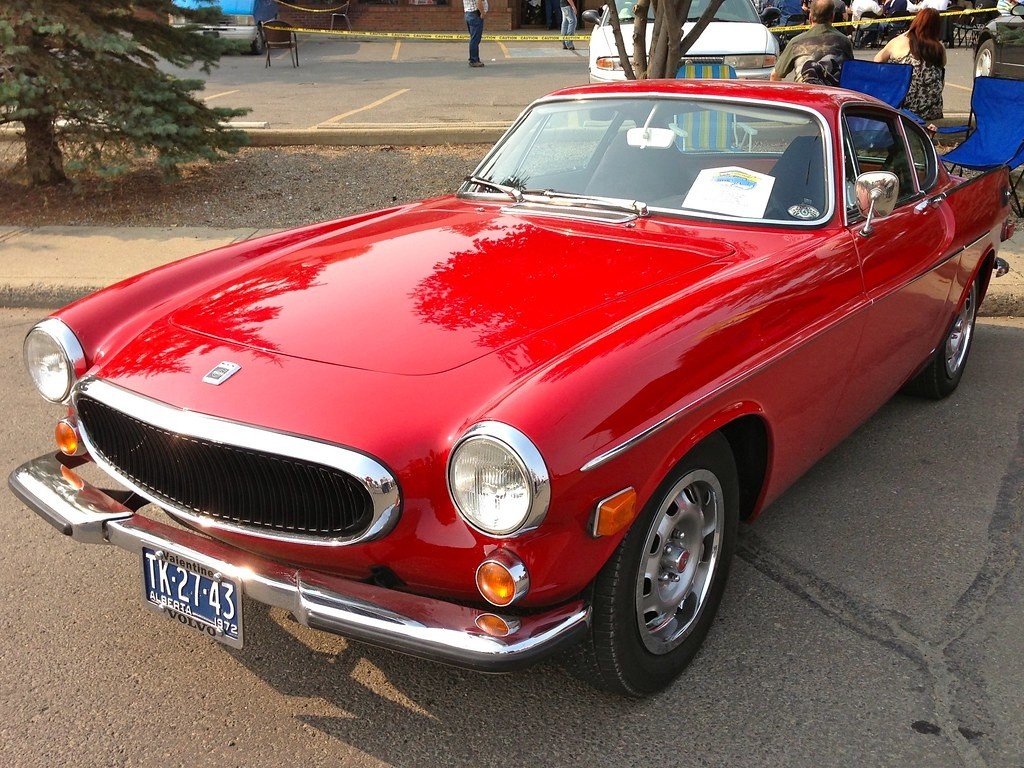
[593,130,694,205]
[330,0,353,31]
[263,20,300,68]
[839,58,927,159]
[926,76,1024,218]
[762,135,825,219]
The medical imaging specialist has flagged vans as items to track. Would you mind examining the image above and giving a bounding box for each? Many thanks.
[169,0,281,55]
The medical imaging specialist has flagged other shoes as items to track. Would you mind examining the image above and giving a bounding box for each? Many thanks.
[568,47,575,50]
[563,45,567,49]
[469,62,484,67]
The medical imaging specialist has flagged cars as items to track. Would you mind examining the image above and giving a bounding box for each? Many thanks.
[7,78,1015,699]
[972,4,1024,84]
[581,1,782,83]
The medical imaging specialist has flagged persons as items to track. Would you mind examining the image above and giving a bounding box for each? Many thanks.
[984,0,1024,24]
[769,0,855,86]
[758,0,954,48]
[543,0,563,31]
[885,178,894,198]
[559,0,578,50]
[463,0,488,67]
[880,178,886,196]
[872,7,947,122]
